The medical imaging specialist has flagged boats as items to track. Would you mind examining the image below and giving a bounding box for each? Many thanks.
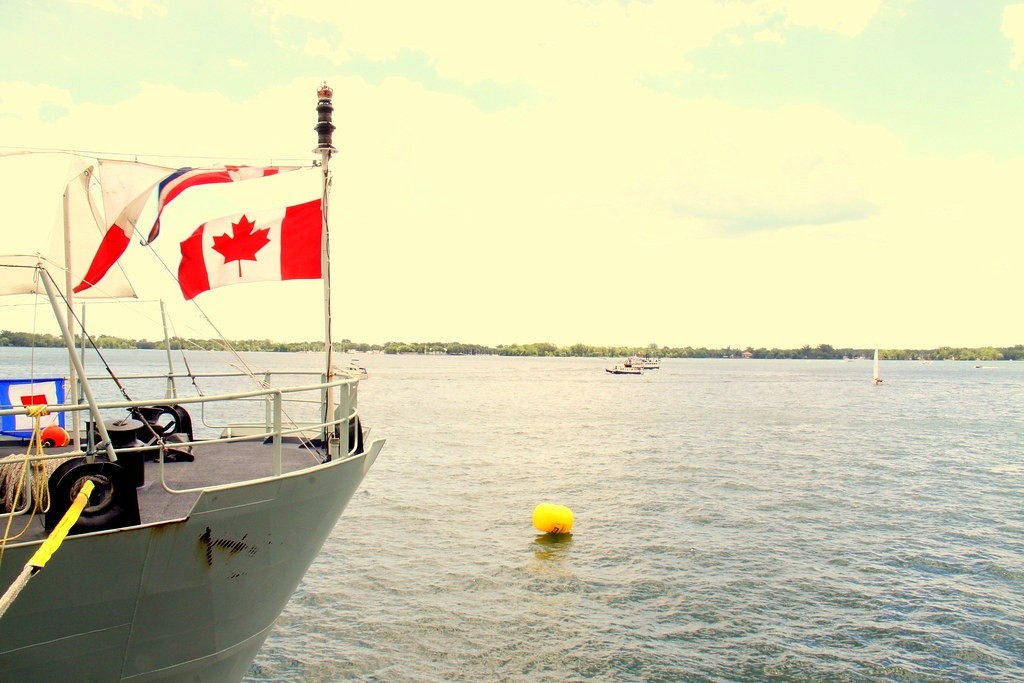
[606,366,642,375]
[335,357,369,382]
[1,82,386,683]
[623,353,660,370]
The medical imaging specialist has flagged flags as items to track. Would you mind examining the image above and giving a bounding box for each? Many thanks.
[71,161,321,303]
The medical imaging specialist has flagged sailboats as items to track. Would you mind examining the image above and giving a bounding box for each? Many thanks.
[975,359,981,369]
[872,350,884,387]
